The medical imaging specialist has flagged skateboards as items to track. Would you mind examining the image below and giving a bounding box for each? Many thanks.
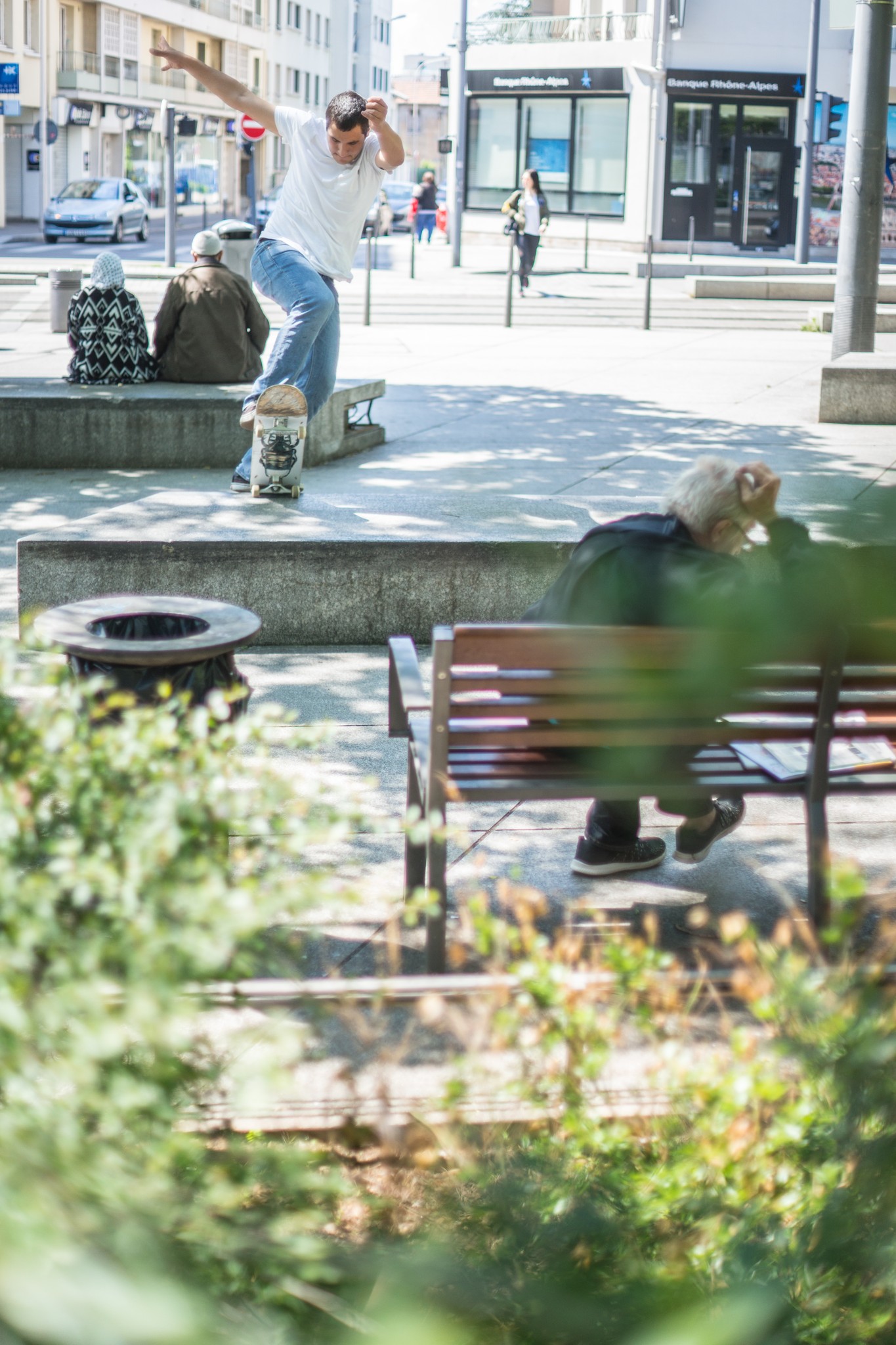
[245,383,311,503]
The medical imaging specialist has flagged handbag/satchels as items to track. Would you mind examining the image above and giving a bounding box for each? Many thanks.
[504,194,521,237]
[412,184,426,200]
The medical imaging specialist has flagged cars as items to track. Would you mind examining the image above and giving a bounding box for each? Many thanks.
[245,188,285,232]
[363,182,449,237]
[46,176,151,243]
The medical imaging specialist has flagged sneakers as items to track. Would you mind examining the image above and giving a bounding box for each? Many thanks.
[674,793,744,861]
[239,403,257,430]
[570,834,666,876]
[230,471,252,492]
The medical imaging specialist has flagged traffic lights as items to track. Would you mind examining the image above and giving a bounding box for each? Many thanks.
[820,94,843,143]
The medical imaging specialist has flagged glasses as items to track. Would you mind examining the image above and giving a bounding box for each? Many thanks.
[726,519,754,553]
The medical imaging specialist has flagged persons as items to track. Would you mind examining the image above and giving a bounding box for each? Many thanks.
[148,26,405,493]
[151,230,271,385]
[495,452,818,879]
[62,249,158,386]
[501,169,550,299]
[378,189,394,236]
[411,171,439,244]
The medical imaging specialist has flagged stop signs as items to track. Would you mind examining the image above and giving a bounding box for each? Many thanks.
[240,114,267,142]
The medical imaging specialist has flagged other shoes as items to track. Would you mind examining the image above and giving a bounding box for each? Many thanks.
[519,286,530,297]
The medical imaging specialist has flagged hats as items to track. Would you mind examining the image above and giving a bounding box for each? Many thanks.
[89,251,125,290]
[192,230,222,256]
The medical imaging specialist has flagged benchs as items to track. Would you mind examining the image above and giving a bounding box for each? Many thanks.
[389,623,895,974]
